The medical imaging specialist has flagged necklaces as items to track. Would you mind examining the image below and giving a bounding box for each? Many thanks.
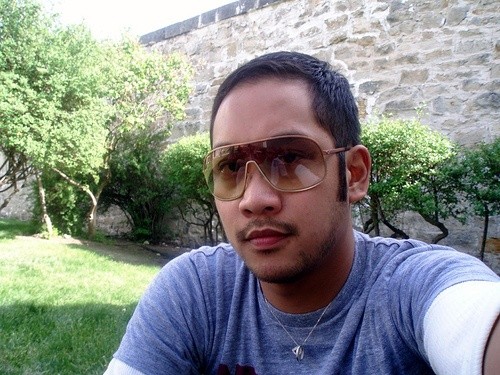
[261,294,330,361]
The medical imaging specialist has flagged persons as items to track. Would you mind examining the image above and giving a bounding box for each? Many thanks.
[102,51,500,375]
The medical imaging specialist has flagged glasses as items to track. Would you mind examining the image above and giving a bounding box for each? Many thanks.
[201,135,352,202]
